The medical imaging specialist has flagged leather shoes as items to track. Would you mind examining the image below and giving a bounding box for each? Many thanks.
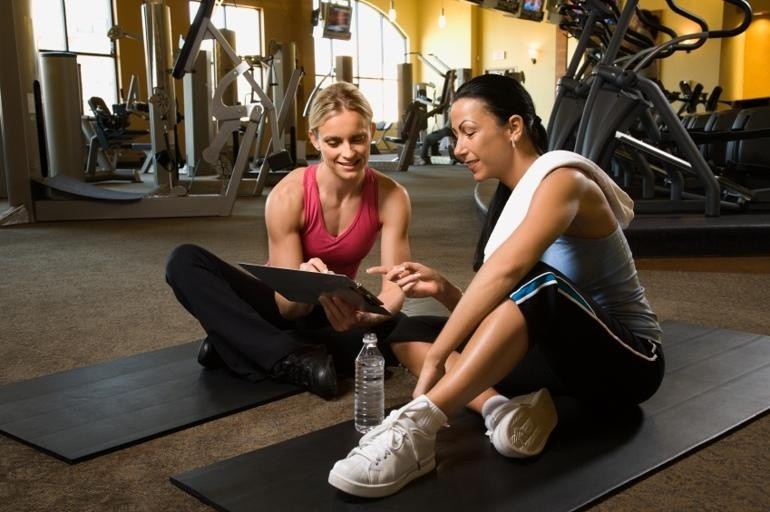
[270,344,338,401]
[198,338,262,379]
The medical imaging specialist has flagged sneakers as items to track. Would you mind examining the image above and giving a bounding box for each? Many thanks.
[328,398,436,498]
[485,388,558,459]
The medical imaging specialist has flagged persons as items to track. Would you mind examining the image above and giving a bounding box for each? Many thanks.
[165,80,414,402]
[328,73,666,500]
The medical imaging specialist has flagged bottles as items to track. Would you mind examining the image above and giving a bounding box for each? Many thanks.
[354,332,385,435]
